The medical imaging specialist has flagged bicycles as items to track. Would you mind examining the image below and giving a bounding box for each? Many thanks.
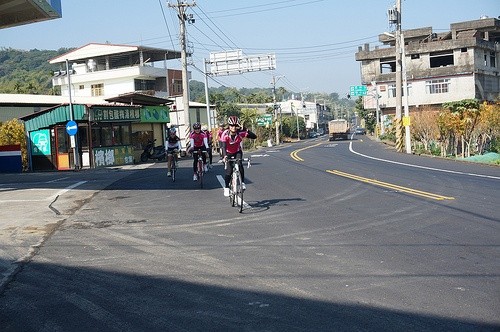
[222,155,252,213]
[166,150,179,182]
[191,150,209,189]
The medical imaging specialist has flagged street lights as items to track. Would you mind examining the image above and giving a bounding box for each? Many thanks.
[384,32,413,154]
[315,95,329,138]
[273,74,285,146]
[296,107,302,142]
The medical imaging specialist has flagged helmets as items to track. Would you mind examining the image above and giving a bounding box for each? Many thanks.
[192,122,201,129]
[169,128,176,132]
[227,116,240,126]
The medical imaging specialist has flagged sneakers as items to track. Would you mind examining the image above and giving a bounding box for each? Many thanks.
[242,183,247,190]
[209,163,214,169]
[204,165,208,172]
[167,172,171,177]
[193,173,198,180]
[223,187,229,197]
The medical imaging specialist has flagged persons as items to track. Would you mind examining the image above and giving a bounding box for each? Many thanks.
[165,128,181,176]
[218,116,256,196]
[190,123,212,180]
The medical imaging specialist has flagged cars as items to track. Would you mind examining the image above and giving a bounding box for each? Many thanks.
[309,132,320,138]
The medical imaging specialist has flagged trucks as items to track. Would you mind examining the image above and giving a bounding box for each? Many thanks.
[328,119,350,140]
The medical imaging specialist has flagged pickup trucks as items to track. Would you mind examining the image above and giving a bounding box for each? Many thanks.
[319,128,324,135]
